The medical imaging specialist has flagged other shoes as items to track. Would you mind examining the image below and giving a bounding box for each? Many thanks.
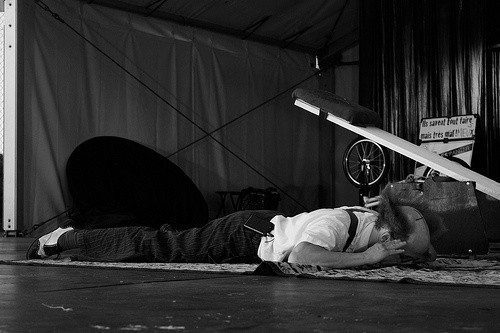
[26,224,75,260]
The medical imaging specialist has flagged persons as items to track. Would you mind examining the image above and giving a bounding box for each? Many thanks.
[25,184,445,271]
